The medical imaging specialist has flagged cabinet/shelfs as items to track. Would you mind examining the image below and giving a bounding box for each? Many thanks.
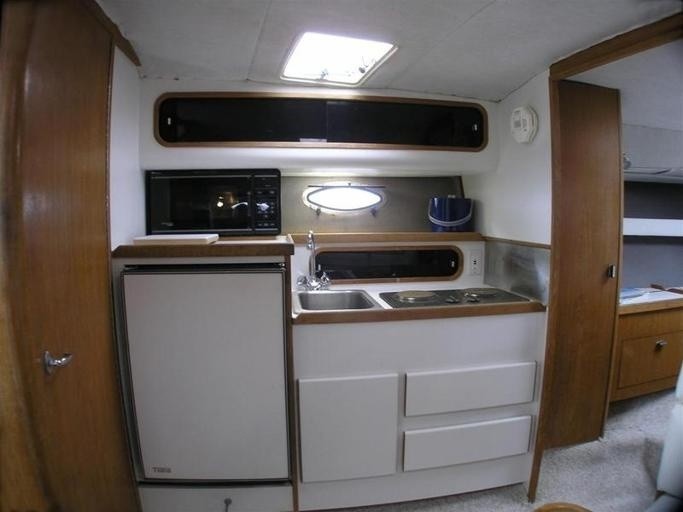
[609,307,683,403]
[622,124,683,184]
[291,311,547,511]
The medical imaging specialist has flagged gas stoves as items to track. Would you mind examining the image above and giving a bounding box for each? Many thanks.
[379,286,539,312]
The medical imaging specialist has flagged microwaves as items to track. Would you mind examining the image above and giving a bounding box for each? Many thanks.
[145,168,282,237]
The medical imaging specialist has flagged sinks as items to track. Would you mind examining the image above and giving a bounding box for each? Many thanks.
[292,290,386,314]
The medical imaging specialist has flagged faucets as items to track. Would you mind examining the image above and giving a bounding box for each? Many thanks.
[306,229,318,278]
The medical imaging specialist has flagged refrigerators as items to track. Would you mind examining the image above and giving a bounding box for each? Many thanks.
[117,268,295,511]
